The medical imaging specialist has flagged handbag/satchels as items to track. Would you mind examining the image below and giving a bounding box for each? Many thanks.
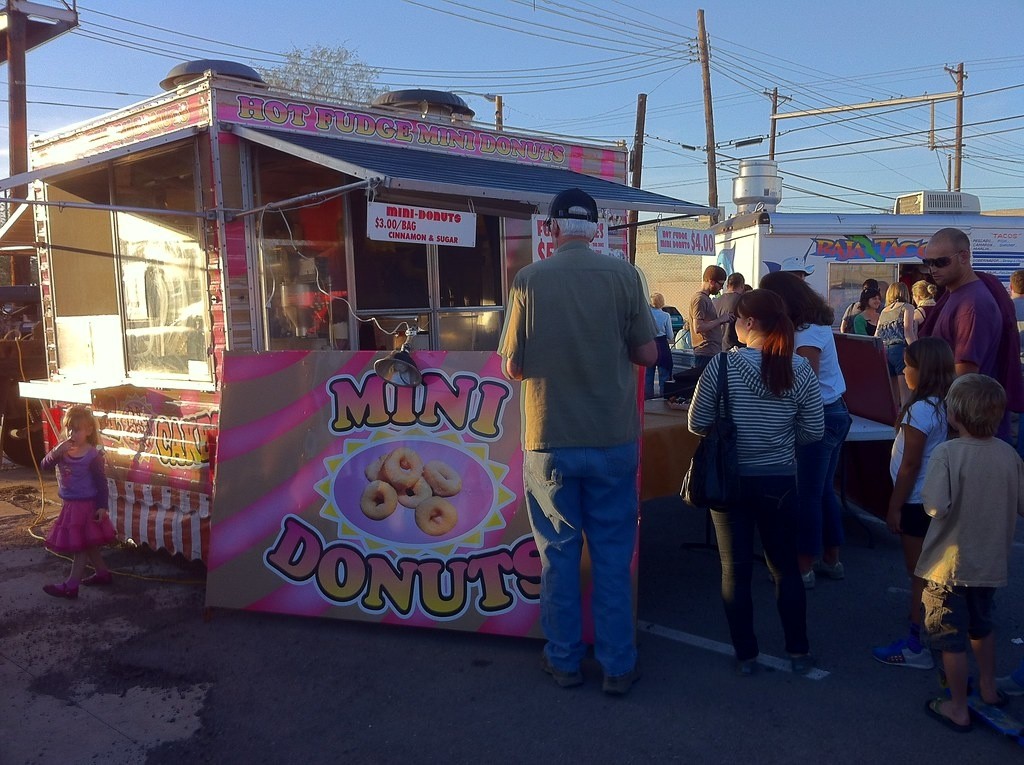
[680,416,737,509]
[844,315,854,334]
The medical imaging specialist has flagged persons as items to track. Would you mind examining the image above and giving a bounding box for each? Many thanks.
[872,337,949,670]
[688,291,824,677]
[500,189,659,696]
[643,265,816,399]
[913,375,1024,735]
[754,273,852,587]
[839,269,1024,373]
[916,228,1024,438]
[40,405,116,599]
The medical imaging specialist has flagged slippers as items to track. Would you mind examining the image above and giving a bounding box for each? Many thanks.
[924,698,975,733]
[982,688,1008,708]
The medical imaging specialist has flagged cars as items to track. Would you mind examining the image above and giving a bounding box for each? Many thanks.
[662,307,684,328]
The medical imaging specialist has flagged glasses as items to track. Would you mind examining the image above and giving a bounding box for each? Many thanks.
[716,281,724,286]
[922,250,970,268]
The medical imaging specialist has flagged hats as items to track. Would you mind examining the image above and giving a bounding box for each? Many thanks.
[779,256,815,276]
[546,188,598,224]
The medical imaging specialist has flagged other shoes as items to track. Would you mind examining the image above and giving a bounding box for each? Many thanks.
[539,654,584,686]
[742,662,758,675]
[603,672,645,695]
[995,675,1024,695]
[802,570,816,588]
[43,582,79,599]
[811,561,845,579]
[792,651,817,674]
[80,572,112,586]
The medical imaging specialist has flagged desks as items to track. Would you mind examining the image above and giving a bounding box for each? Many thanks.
[841,414,898,440]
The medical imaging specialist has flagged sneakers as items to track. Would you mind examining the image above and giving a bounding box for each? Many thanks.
[872,640,934,670]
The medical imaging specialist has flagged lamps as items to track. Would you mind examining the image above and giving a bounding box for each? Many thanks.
[375,325,422,387]
